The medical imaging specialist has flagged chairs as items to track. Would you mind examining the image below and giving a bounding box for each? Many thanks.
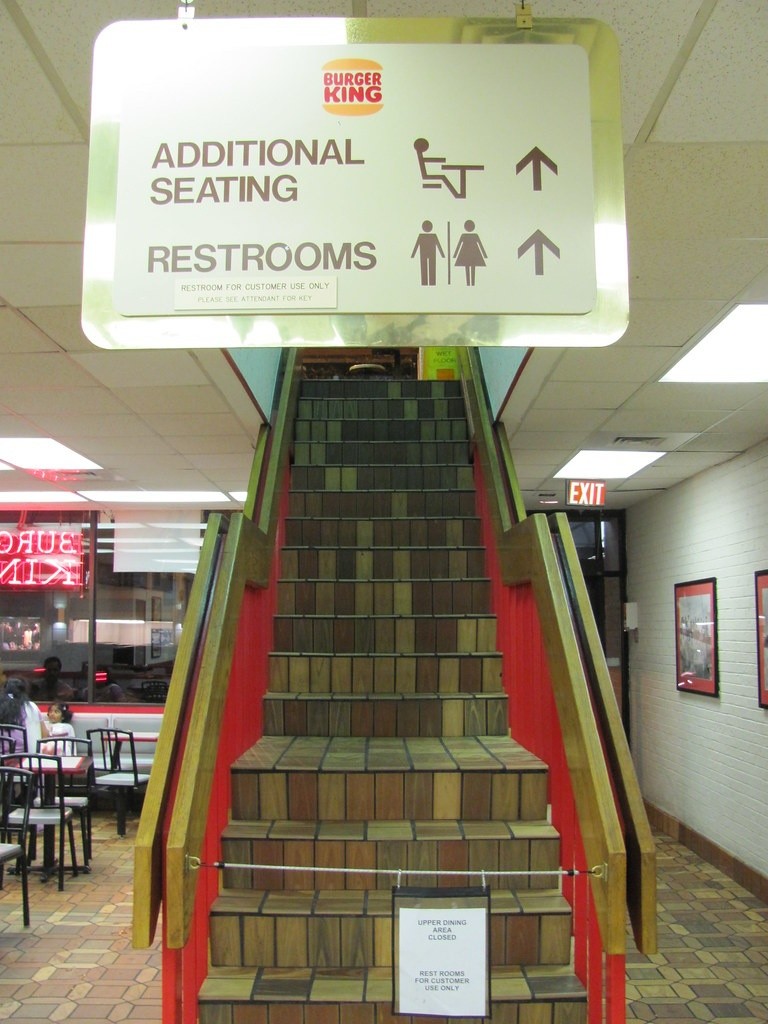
[141,680,168,703]
[0,724,151,927]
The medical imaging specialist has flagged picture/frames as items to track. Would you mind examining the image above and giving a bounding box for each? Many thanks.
[152,596,161,658]
[674,577,718,699]
[755,569,768,710]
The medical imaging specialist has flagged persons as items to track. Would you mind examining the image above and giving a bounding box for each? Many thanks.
[0,675,78,808]
[27,656,126,703]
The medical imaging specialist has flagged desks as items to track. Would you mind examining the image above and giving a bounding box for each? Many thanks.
[4,756,93,883]
[128,685,167,691]
[126,692,167,700]
[103,732,160,770]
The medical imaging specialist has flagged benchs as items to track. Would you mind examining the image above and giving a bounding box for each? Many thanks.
[41,712,164,778]
